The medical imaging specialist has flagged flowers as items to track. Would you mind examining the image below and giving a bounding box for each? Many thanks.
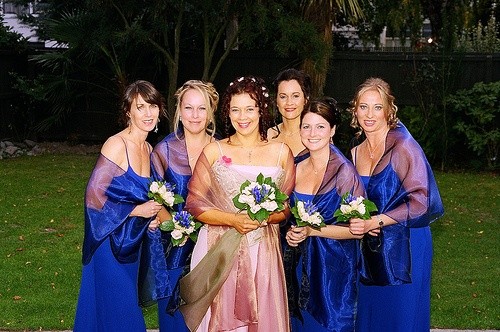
[146,180,184,209]
[231,172,289,227]
[158,210,201,248]
[292,200,328,232]
[336,192,377,225]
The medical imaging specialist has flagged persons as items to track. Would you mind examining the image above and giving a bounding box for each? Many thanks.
[147,80,221,332]
[345,78,444,332]
[279,96,368,332]
[177,74,296,332]
[267,69,312,157]
[73,80,170,332]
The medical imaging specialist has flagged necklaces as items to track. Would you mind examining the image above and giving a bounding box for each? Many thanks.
[313,168,322,175]
[241,141,261,164]
[369,129,389,157]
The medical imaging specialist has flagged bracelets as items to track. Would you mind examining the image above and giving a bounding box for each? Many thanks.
[377,214,384,228]
